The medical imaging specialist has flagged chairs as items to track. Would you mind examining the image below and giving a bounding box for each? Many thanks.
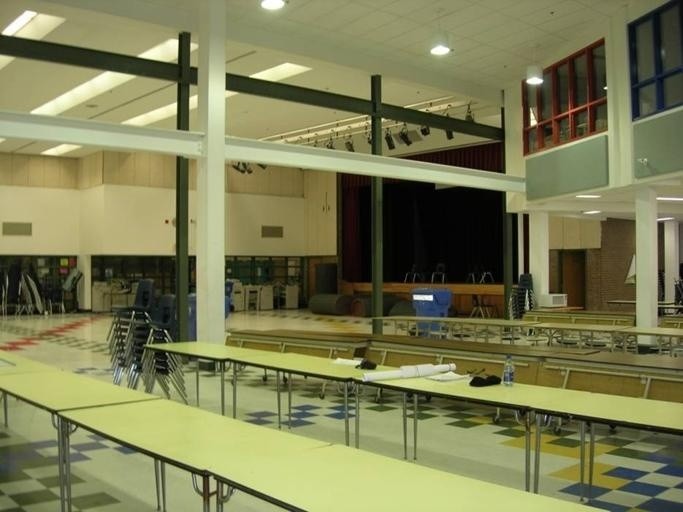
[465,262,495,284]
[105,277,188,404]
[469,294,498,319]
[431,262,448,283]
[508,274,537,320]
[404,263,424,283]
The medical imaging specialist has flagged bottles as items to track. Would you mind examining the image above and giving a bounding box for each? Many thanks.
[503,354,515,388]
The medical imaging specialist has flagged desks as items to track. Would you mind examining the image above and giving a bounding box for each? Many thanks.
[58,397,335,512]
[205,442,614,512]
[1,348,62,401]
[143,314,682,446]
[532,392,682,501]
[1,369,163,511]
[352,368,591,501]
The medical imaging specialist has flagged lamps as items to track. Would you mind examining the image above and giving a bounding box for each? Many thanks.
[384,102,474,150]
[233,161,267,174]
[324,129,372,152]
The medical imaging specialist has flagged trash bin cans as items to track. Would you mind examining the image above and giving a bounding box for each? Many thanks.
[187,294,196,341]
[412,289,451,336]
